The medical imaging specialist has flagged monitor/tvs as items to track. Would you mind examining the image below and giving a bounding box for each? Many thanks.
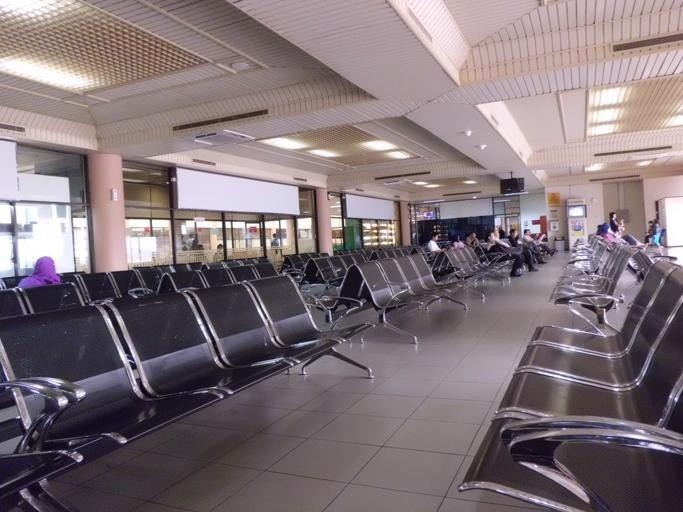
[499,177,525,194]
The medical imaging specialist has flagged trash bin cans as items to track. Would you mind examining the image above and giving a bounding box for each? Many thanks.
[554,236,564,252]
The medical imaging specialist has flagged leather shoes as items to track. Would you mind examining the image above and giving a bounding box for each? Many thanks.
[529,267,539,272]
[539,260,548,264]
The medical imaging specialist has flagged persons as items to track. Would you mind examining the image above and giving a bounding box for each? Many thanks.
[651,218,664,253]
[213,244,224,261]
[189,234,198,250]
[180,233,187,251]
[485,232,539,277]
[645,219,656,247]
[14,256,62,287]
[609,211,624,235]
[451,234,466,248]
[428,232,442,251]
[271,233,280,246]
[598,223,649,253]
[524,229,558,264]
[508,228,546,264]
[197,243,203,251]
[465,231,479,246]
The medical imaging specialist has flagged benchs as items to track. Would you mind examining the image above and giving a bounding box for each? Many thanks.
[133,262,204,273]
[428,250,455,282]
[129,273,167,297]
[1,381,82,512]
[479,244,518,283]
[166,262,281,292]
[287,252,331,268]
[54,267,163,304]
[308,264,373,329]
[311,253,368,287]
[564,241,609,275]
[1,282,87,317]
[551,244,639,333]
[287,259,323,287]
[1,274,376,512]
[441,247,505,302]
[205,256,271,269]
[374,248,412,260]
[631,247,678,280]
[354,253,468,344]
[458,260,683,511]
